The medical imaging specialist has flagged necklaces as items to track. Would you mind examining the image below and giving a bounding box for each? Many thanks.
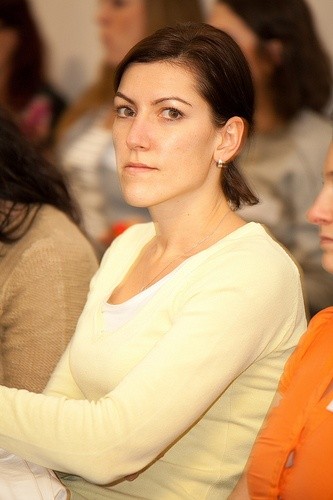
[136,209,233,294]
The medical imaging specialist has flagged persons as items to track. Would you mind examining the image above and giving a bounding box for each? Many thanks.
[0,0,68,150]
[0,108,100,394]
[205,0,333,326]
[1,24,308,500]
[46,0,207,266]
[226,138,333,500]
